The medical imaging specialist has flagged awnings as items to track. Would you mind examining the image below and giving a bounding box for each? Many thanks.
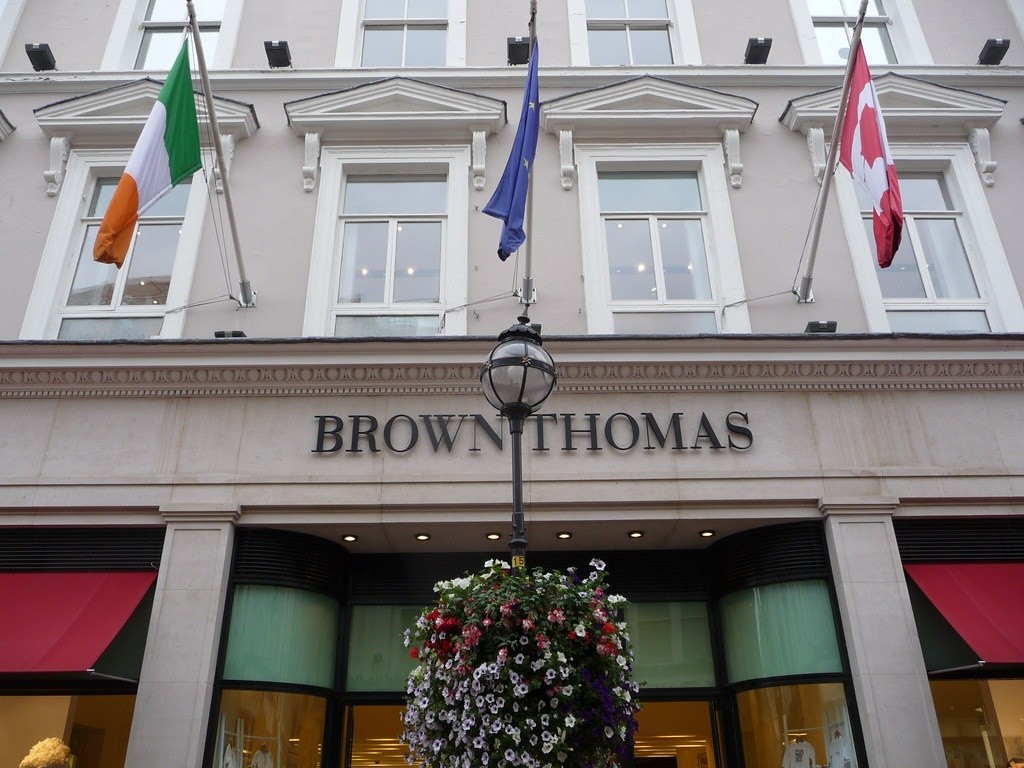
[904,558,1024,680]
[1,566,157,683]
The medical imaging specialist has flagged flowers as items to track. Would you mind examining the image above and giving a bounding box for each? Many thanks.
[398,558,644,768]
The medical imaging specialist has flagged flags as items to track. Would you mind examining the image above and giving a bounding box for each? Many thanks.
[93,40,219,263]
[834,36,906,274]
[479,25,546,265]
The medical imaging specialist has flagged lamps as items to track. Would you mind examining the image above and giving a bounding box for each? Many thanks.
[506,36,531,68]
[743,37,772,65]
[976,38,1010,66]
[25,42,60,73]
[263,41,293,69]
